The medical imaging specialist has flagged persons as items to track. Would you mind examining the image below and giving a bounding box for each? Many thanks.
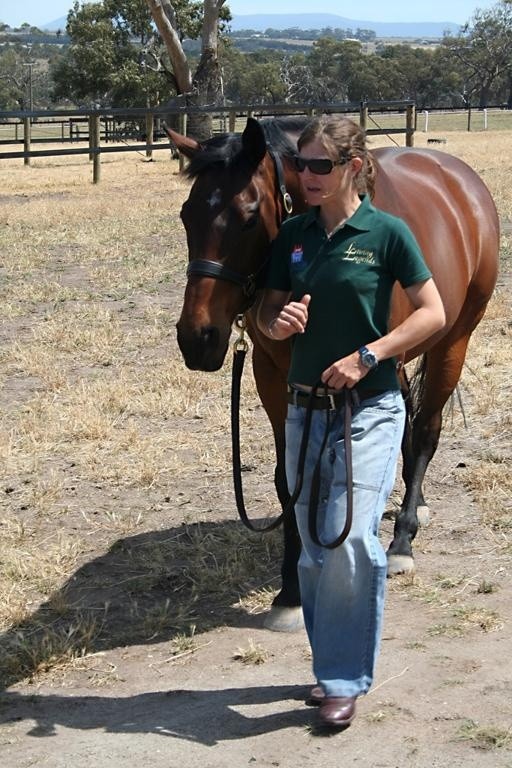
[254,109,449,729]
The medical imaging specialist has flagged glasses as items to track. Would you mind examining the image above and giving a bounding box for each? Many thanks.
[292,154,350,175]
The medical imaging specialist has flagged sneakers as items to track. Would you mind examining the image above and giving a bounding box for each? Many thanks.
[310,679,356,727]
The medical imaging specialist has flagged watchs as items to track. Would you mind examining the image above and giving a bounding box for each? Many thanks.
[358,346,378,370]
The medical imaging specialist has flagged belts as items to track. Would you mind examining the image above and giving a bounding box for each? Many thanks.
[283,383,384,412]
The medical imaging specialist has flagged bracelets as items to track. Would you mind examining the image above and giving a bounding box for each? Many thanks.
[267,311,278,335]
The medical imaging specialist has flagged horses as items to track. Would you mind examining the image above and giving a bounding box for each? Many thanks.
[161,116,501,633]
